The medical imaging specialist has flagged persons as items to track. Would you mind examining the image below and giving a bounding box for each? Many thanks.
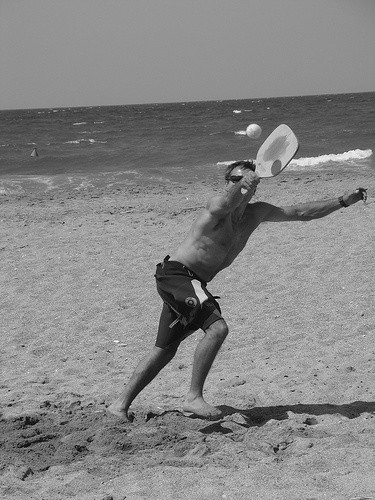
[107,160,368,422]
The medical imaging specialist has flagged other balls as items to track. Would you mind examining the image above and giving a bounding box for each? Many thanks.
[246,123,262,140]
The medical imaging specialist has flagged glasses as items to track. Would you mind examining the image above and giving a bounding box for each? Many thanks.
[225,175,243,183]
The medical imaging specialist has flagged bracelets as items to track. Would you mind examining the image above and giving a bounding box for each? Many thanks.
[337,195,350,208]
